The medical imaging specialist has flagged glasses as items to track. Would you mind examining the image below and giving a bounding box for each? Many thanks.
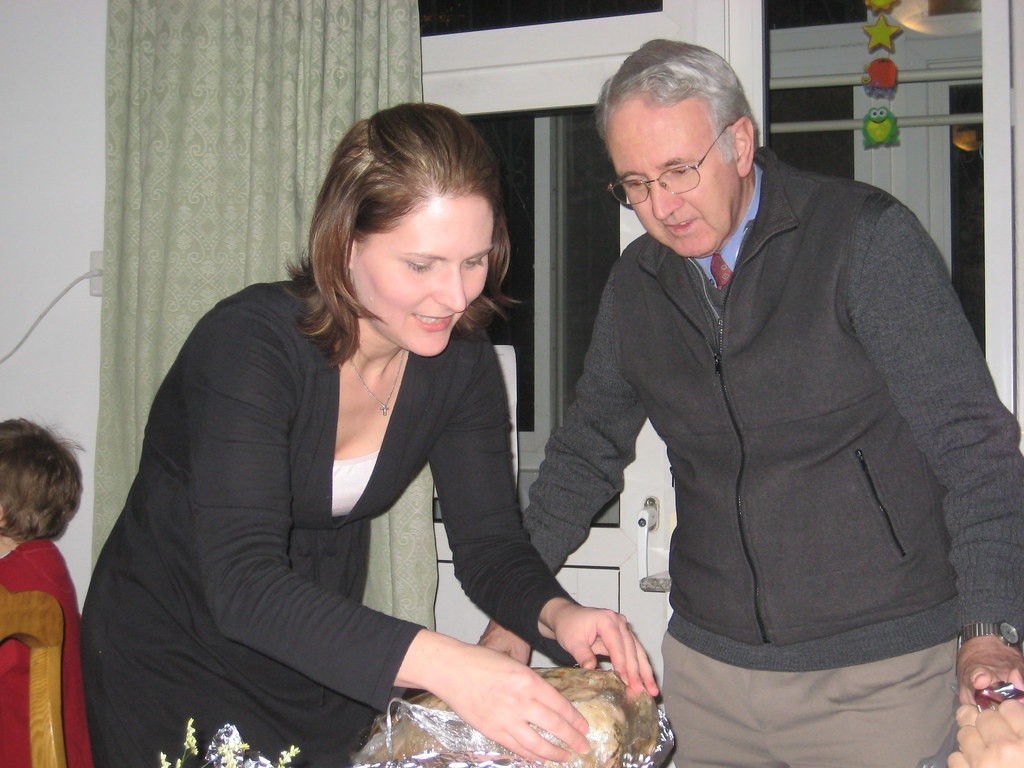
[608,123,733,205]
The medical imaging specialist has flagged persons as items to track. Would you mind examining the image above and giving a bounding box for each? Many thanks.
[81,103,659,768]
[477,38,1024,768]
[0,418,93,768]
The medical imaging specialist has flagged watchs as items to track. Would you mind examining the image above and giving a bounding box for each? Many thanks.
[957,621,1022,650]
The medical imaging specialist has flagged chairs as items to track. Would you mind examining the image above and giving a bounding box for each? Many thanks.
[0,590,67,768]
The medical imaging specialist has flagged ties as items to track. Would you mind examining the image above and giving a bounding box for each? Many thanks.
[711,254,732,290]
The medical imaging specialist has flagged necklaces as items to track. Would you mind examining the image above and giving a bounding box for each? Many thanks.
[352,350,405,416]
